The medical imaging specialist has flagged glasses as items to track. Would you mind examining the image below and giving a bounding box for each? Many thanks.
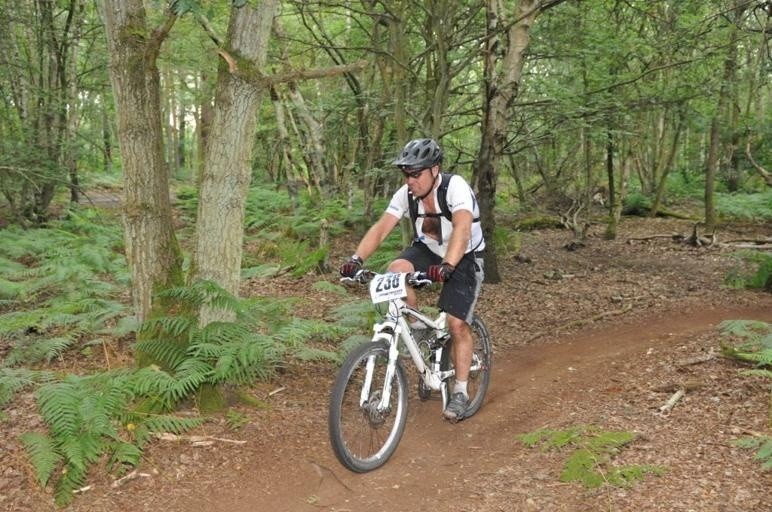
[401,169,424,178]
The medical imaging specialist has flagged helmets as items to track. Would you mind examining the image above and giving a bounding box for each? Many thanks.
[391,137,442,169]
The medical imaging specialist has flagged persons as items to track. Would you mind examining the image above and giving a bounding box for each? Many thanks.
[337,136,486,419]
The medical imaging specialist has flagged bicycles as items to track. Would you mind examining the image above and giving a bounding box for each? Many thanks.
[329,269,492,473]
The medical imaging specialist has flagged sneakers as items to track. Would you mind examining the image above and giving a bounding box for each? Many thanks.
[442,392,471,422]
[402,325,433,360]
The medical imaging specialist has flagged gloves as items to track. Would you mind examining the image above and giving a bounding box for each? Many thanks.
[427,261,454,284]
[337,256,363,277]
